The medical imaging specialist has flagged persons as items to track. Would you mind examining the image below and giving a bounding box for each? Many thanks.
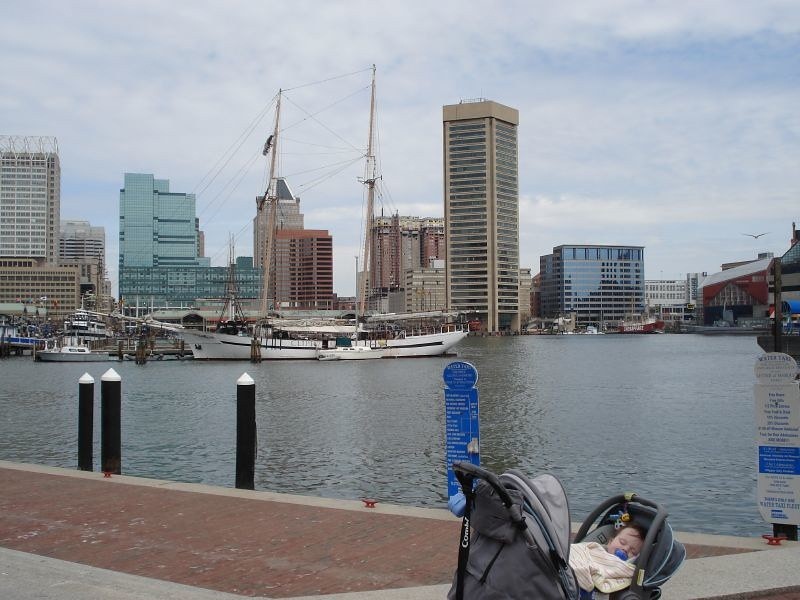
[569,524,646,594]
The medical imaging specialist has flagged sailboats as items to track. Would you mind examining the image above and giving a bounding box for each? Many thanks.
[12,64,470,362]
